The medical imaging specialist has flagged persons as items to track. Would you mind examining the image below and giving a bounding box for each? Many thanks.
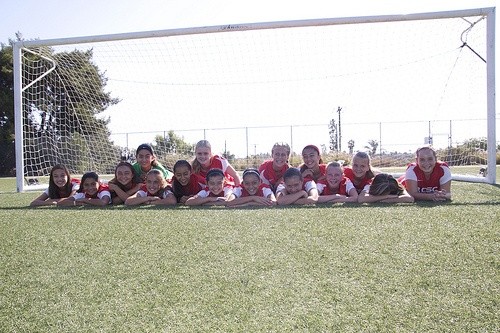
[30,163,81,206]
[316,162,359,203]
[108,143,174,187]
[342,151,375,193]
[297,145,327,181]
[357,173,414,203]
[167,159,208,203]
[111,161,145,204]
[257,140,293,195]
[397,146,452,203]
[226,168,277,207]
[275,167,318,206]
[57,171,112,207]
[191,140,241,187]
[124,169,178,206]
[185,168,233,206]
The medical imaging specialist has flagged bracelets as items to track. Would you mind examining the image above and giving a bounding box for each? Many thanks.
[73,200,76,206]
[150,200,151,204]
